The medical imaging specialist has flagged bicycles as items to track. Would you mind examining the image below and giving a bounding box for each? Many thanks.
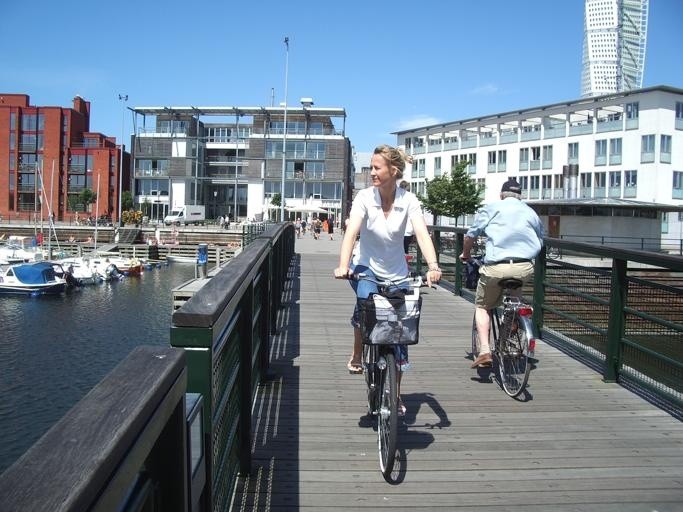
[337,275,439,478]
[463,255,535,398]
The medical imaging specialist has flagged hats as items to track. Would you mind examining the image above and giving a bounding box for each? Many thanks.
[502,180,521,194]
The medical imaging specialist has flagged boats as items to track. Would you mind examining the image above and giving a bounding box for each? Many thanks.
[0,245,144,295]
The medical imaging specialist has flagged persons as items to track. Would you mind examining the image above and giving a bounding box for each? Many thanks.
[458,179,546,369]
[293,217,335,241]
[218,215,224,230]
[223,214,229,230]
[332,141,445,376]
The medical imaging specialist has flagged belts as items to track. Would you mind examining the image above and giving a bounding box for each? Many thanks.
[479,255,531,267]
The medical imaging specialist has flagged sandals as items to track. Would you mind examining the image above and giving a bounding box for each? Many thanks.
[346,348,364,375]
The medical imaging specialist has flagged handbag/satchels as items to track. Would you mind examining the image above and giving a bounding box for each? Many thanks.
[370,277,423,345]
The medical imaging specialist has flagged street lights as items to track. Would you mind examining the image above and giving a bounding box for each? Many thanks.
[282,37,289,220]
[119,95,128,227]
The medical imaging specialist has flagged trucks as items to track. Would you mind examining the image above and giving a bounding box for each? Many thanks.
[165,205,205,226]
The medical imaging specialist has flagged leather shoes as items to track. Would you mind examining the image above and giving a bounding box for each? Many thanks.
[469,353,494,369]
